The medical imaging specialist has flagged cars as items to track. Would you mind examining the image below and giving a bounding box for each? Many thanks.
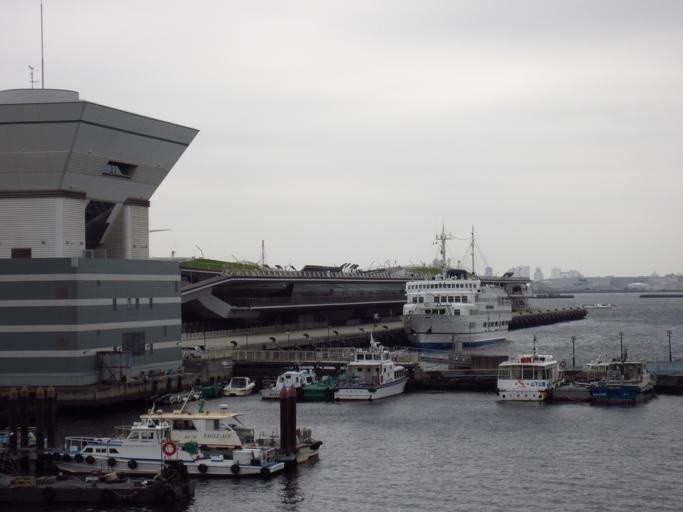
[180,345,203,353]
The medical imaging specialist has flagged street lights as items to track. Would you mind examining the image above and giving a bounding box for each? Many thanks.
[371,312,380,334]
[570,334,577,367]
[284,327,292,348]
[666,329,672,361]
[617,330,624,359]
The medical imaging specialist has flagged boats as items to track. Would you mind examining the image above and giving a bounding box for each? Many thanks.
[108,386,323,462]
[0,438,204,508]
[222,375,257,396]
[46,408,285,482]
[401,228,514,347]
[258,360,317,398]
[331,331,412,405]
[577,302,617,310]
[493,351,565,403]
[589,361,658,406]
[527,288,576,299]
